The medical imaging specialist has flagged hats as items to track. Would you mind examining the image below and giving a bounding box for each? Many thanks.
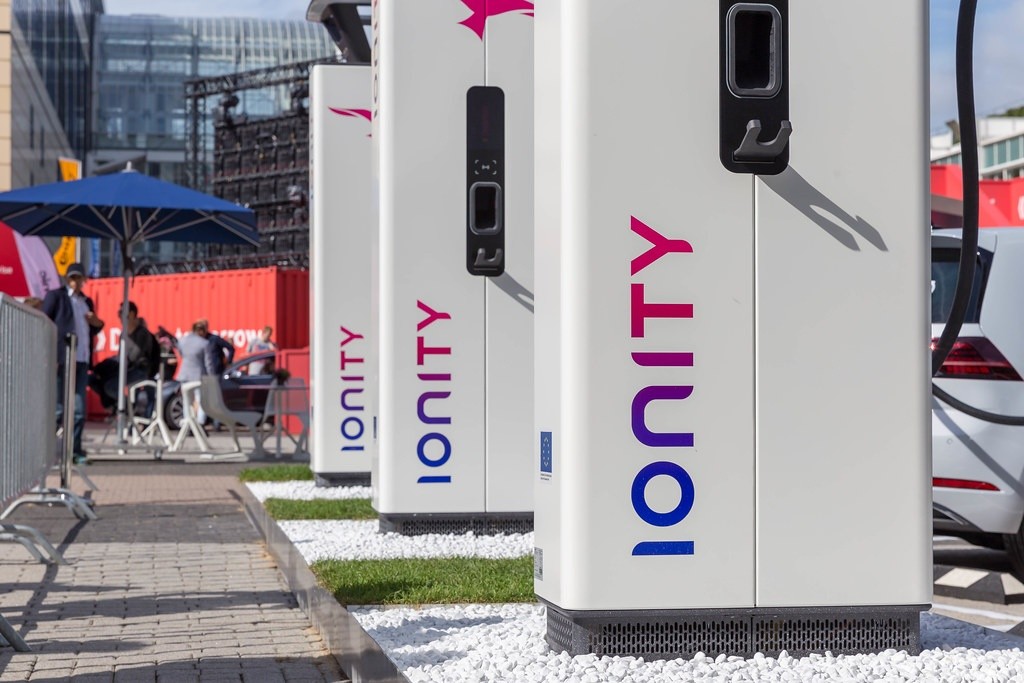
[66,262,85,277]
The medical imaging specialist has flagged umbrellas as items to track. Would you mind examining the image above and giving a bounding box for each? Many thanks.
[0,168,260,442]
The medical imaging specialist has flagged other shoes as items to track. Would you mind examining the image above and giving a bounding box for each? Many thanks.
[189,424,210,438]
[74,456,88,464]
[263,423,272,431]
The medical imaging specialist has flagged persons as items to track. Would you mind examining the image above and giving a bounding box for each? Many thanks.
[88,301,160,425]
[175,320,234,437]
[39,263,104,462]
[246,326,276,376]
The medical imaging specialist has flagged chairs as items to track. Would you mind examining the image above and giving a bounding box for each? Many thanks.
[200,374,265,453]
[251,377,311,455]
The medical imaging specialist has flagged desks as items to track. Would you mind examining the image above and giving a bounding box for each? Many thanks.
[240,383,310,459]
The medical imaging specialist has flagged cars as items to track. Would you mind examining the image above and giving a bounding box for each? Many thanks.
[161,351,278,436]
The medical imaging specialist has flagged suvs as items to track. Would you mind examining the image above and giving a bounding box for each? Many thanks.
[931,225,1023,583]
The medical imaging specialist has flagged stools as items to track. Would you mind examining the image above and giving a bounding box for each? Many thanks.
[97,366,170,461]
